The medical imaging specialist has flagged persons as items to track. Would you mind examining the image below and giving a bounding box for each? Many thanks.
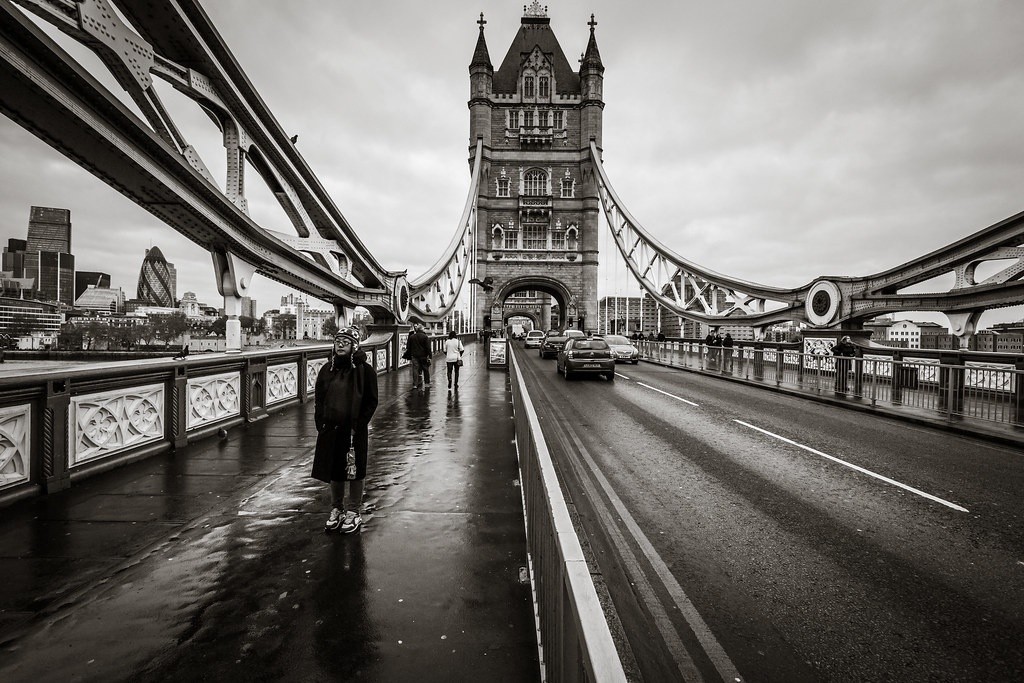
[402,323,433,388]
[632,329,665,354]
[310,324,379,533]
[706,330,734,372]
[831,336,856,392]
[479,328,506,343]
[444,332,464,389]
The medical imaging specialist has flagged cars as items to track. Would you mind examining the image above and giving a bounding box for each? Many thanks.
[524,330,545,349]
[547,331,560,336]
[556,336,616,381]
[602,335,639,364]
[563,330,585,339]
[519,333,527,341]
[538,335,569,359]
[512,332,521,340]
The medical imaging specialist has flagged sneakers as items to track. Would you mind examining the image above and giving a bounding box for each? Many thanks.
[325,507,345,529]
[342,510,363,534]
[425,381,433,388]
[412,385,417,388]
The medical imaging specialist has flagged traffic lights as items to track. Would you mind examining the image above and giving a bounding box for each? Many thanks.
[568,317,573,327]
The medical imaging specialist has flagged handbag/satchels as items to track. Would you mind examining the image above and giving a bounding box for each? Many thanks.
[457,359,463,366]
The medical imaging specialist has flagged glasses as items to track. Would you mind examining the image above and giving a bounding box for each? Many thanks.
[332,339,351,346]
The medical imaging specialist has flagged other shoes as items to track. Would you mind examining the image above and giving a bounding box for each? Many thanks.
[448,380,452,388]
[454,383,459,387]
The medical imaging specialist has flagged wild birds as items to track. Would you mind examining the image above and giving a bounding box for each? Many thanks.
[175,345,189,359]
[290,134,298,144]
[218,426,227,439]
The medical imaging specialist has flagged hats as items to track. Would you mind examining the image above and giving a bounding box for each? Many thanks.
[330,326,360,371]
